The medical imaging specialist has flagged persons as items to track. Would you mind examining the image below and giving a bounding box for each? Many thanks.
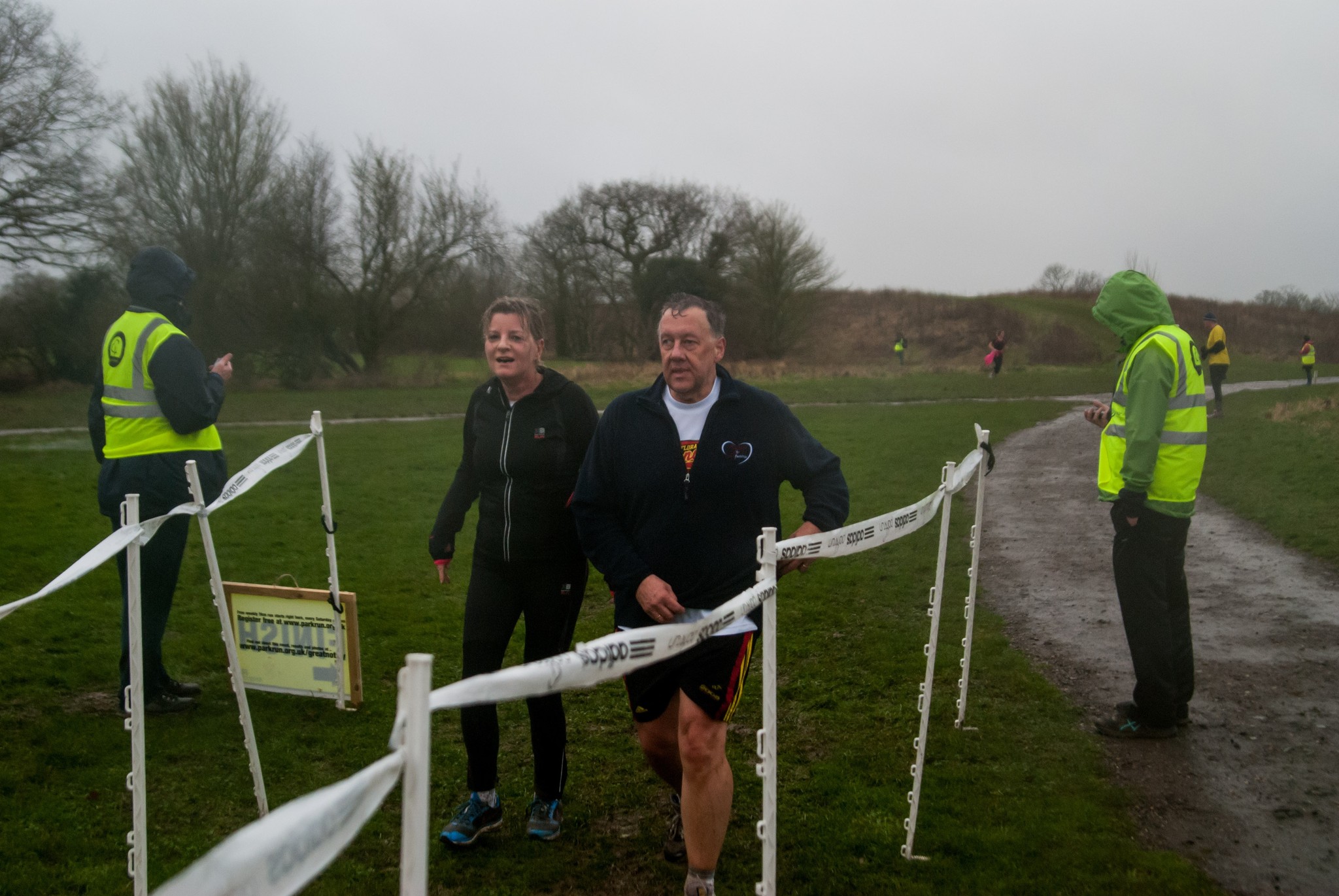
[1298,336,1316,383]
[988,330,1006,379]
[86,246,234,720]
[894,331,906,366]
[1083,271,1208,738]
[567,291,850,896]
[429,297,600,845]
[1199,310,1231,418]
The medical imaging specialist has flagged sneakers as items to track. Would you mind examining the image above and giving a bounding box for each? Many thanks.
[525,794,561,840]
[1094,715,1178,740]
[146,695,195,714]
[439,793,503,845]
[1117,701,1189,725]
[164,678,202,695]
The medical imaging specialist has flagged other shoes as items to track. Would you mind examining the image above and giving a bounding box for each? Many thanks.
[665,794,684,840]
